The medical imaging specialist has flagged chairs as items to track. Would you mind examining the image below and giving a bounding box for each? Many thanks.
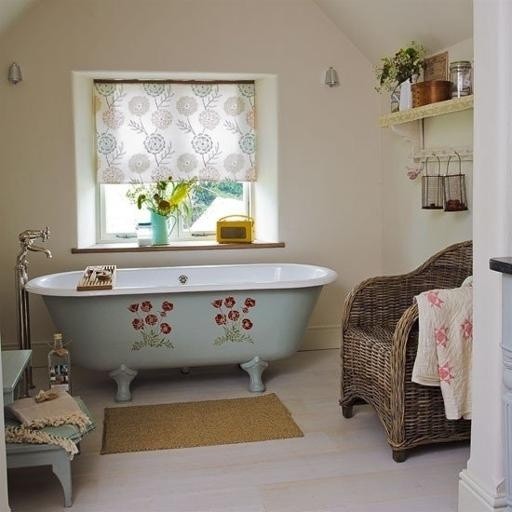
[339,239,472,462]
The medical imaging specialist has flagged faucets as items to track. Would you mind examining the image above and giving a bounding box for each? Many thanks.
[28,242,53,260]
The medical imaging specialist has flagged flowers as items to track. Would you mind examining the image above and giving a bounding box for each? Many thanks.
[124,168,199,237]
[374,41,427,97]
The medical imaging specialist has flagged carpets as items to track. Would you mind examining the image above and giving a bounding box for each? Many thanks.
[100,394,305,456]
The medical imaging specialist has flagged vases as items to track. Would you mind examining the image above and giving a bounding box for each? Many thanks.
[149,207,176,246]
[398,74,417,112]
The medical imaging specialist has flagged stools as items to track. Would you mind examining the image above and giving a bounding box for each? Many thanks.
[6,400,79,507]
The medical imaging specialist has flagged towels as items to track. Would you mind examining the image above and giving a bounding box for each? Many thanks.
[412,276,472,422]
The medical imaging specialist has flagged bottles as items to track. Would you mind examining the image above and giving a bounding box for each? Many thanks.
[449,59,472,99]
[136,222,154,249]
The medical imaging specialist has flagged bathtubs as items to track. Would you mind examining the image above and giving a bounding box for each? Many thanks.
[24,262,337,403]
